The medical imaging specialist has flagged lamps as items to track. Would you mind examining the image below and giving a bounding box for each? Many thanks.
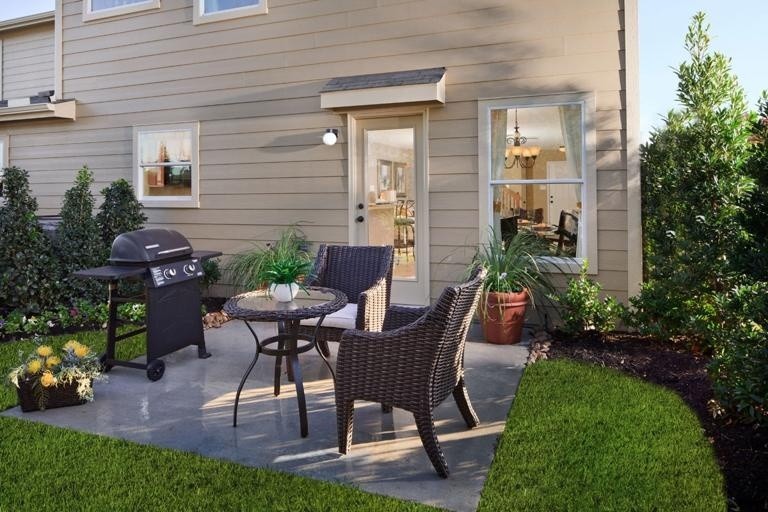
[504,108,539,168]
[322,129,338,146]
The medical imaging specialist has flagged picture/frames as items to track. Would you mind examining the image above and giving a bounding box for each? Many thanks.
[377,159,393,198]
[393,162,408,197]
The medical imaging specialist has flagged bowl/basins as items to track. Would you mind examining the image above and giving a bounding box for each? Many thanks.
[532,225,552,231]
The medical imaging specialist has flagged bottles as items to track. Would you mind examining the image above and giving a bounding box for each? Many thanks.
[164,159,171,185]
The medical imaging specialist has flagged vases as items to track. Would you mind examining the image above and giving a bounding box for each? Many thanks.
[16,372,93,412]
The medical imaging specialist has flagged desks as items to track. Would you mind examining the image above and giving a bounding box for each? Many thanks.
[222,286,349,438]
[516,218,570,250]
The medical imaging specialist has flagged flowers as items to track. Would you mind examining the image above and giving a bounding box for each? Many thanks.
[8,340,109,413]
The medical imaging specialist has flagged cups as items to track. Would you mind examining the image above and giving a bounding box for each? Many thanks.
[147,171,157,186]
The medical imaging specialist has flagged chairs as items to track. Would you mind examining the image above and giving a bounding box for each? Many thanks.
[394,200,416,265]
[283,243,393,382]
[335,265,488,479]
[510,207,543,225]
[554,209,578,257]
[500,217,518,252]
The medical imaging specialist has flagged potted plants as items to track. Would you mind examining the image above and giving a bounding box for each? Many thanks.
[223,220,312,290]
[248,255,320,303]
[438,225,583,345]
